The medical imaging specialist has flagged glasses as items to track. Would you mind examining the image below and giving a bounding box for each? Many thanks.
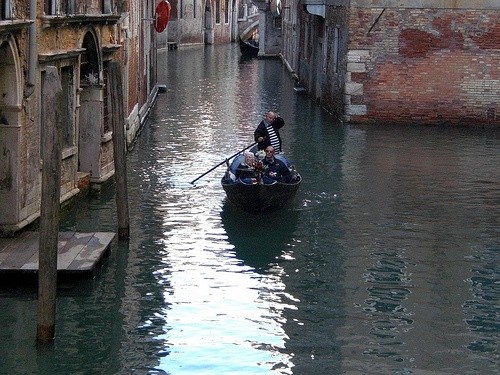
[266,149,273,153]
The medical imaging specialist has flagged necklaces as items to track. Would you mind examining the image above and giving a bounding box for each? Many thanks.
[245,163,251,168]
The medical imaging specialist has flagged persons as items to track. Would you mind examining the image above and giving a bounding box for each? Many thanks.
[254,111,285,154]
[260,145,295,184]
[236,152,259,184]
[249,34,259,46]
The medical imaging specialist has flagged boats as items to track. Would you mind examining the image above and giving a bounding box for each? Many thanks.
[221,143,303,214]
[238,35,259,53]
